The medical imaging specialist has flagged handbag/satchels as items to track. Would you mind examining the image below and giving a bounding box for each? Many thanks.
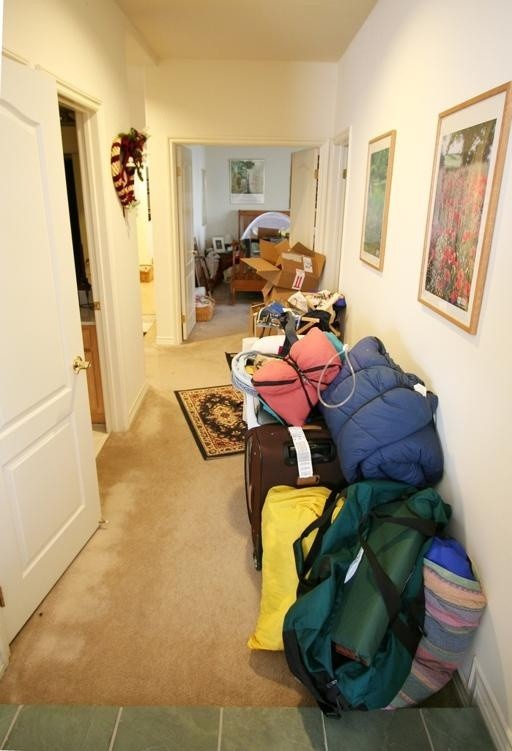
[282,480,453,720]
[246,486,346,652]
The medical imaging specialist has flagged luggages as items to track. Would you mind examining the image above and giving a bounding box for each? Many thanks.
[244,423,342,571]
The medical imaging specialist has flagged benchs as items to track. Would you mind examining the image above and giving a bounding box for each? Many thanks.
[231,209,290,265]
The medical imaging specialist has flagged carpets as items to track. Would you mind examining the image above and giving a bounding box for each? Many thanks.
[173,383,248,460]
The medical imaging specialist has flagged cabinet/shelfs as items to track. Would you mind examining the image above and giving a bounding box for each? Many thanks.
[82,325,105,424]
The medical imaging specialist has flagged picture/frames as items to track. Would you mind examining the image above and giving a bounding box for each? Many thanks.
[359,130,397,272]
[212,237,225,254]
[419,80,512,335]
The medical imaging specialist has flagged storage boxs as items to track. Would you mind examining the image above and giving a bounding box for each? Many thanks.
[240,238,326,337]
